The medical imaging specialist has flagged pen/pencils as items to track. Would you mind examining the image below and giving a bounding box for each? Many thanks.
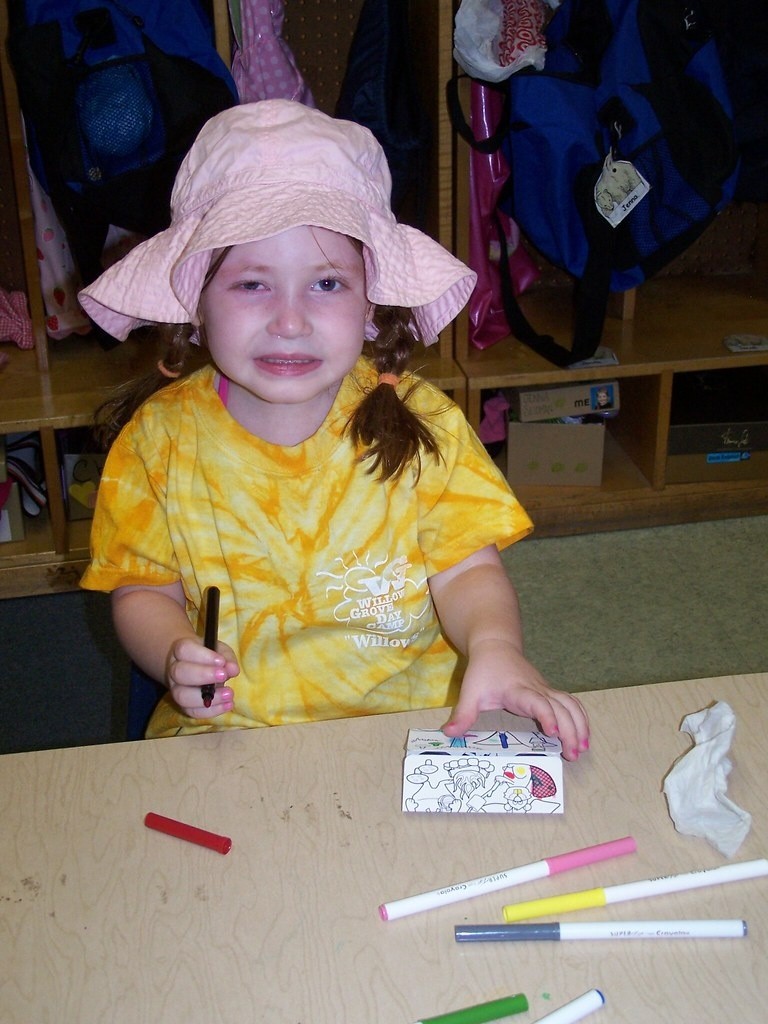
[415,987,609,1024]
[378,835,637,921]
[501,855,768,924]
[453,916,748,945]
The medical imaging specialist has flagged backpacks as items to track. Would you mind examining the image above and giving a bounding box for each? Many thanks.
[7,0,241,236]
[446,0,741,370]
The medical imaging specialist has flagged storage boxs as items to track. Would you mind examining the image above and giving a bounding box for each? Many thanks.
[667,421,768,482]
[507,382,620,487]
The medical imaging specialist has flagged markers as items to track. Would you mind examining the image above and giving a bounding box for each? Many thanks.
[201,585,223,709]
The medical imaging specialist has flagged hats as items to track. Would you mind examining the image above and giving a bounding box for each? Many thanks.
[77,99,478,347]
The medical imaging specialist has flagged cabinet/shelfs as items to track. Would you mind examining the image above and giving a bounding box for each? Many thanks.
[0,0,768,597]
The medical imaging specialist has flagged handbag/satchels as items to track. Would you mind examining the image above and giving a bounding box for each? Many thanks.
[338,0,433,233]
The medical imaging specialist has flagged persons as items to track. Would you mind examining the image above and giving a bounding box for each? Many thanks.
[594,388,613,410]
[78,97,591,763]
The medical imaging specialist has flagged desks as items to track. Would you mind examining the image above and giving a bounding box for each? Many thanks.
[1,673,768,1024]
